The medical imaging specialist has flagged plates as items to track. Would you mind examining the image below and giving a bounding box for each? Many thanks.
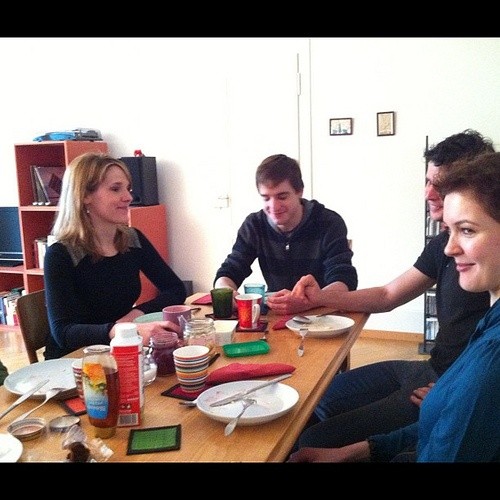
[134,312,162,322]
[3,358,77,398]
[197,380,300,426]
[284,315,356,338]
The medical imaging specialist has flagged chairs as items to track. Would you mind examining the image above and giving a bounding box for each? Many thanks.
[14,288,50,364]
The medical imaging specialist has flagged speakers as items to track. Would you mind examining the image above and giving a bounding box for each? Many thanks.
[120,156,159,207]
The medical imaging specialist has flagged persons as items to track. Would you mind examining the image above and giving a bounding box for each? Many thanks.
[44,153,187,364]
[285,128,500,463]
[212,154,359,316]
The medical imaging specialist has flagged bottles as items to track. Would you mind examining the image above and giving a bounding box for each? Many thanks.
[110,322,145,428]
[81,345,119,439]
[182,318,217,360]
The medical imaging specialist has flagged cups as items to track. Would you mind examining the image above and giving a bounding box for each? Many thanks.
[244,284,265,312]
[235,293,263,330]
[71,358,86,409]
[211,288,233,318]
[163,306,191,329]
[173,345,210,392]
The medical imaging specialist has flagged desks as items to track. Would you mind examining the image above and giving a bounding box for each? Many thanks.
[0,292,371,464]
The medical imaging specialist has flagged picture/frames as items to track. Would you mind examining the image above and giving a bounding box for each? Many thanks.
[377,112,394,136]
[329,118,352,136]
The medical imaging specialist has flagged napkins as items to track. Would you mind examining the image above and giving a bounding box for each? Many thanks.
[272,316,293,330]
[207,363,297,386]
[190,294,213,305]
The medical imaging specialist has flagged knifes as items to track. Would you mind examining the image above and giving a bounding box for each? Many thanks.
[209,373,292,406]
[0,380,49,419]
[316,311,343,317]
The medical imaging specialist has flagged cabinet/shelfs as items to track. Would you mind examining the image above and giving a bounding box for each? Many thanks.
[0,141,170,332]
[418,136,447,355]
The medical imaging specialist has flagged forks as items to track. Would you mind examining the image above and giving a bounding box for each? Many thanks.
[224,399,257,437]
[6,387,67,428]
[297,328,308,358]
[259,331,269,339]
[178,385,209,406]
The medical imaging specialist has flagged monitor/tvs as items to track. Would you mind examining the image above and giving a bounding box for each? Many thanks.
[0,207,24,266]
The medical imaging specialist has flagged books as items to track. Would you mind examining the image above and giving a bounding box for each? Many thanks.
[0,285,25,326]
[30,165,66,206]
[425,209,442,339]
